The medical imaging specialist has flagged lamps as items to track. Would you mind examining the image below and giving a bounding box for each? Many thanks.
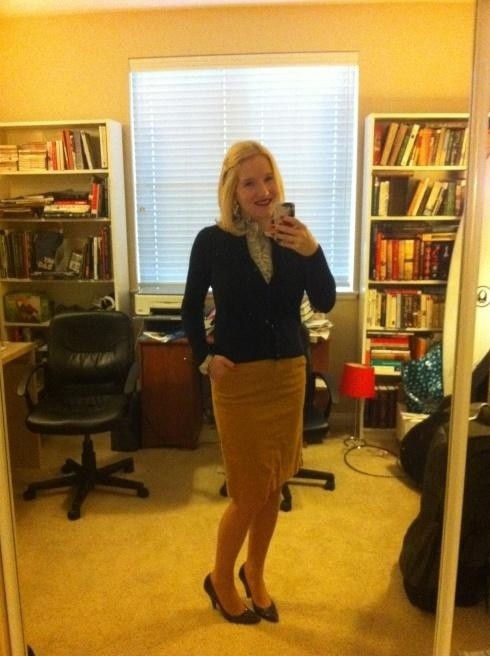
[339,363,378,448]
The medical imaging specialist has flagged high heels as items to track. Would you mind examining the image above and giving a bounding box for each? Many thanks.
[204,572,261,625]
[238,563,279,623]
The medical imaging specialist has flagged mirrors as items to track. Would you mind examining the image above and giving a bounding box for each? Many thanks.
[1,4,488,656]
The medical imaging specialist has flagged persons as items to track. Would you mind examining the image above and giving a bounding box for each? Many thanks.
[181,140,336,624]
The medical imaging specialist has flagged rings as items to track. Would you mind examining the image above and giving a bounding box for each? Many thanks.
[289,235,297,243]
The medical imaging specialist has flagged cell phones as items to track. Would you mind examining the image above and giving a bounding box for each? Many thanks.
[275,202,294,240]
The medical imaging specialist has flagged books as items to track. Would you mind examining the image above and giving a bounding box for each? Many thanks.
[0,126,111,281]
[363,122,470,428]
[300,296,335,334]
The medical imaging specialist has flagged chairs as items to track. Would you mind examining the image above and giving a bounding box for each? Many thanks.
[15,312,150,520]
[220,324,334,511]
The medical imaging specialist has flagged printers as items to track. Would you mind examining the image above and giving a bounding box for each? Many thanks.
[133,286,184,321]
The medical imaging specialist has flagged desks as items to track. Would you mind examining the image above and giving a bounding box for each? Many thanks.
[2,342,42,470]
[138,316,329,449]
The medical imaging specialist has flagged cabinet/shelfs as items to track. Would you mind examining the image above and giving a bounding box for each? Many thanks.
[3,119,131,340]
[357,112,468,440]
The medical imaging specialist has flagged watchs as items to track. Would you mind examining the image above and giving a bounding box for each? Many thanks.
[198,353,213,376]
